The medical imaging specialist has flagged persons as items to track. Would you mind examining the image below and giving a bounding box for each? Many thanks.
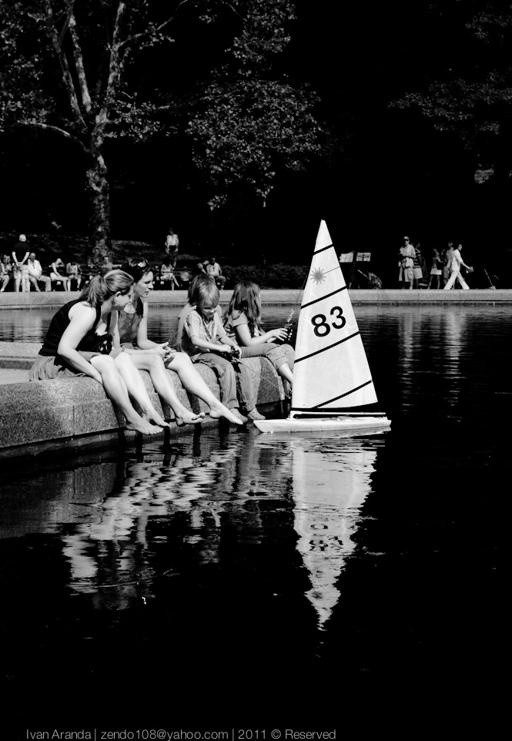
[0,228,226,294]
[116,267,244,430]
[175,273,223,354]
[29,269,171,438]
[396,236,474,290]
[181,279,266,424]
[221,280,294,421]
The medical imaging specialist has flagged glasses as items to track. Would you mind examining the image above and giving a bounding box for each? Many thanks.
[137,258,149,269]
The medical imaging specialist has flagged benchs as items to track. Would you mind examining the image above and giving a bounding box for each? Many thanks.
[0,259,226,292]
